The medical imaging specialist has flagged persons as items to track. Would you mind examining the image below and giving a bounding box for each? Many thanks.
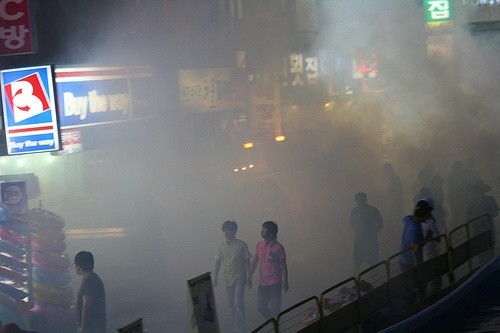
[349,192,383,275]
[414,160,483,230]
[383,163,403,230]
[417,196,441,263]
[400,201,434,273]
[212,219,252,333]
[73,250,106,333]
[466,177,499,265]
[250,220,290,333]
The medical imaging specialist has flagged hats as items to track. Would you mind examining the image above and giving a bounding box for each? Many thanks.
[466,179,493,192]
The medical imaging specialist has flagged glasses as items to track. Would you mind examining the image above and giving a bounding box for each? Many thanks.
[73,263,75,267]
